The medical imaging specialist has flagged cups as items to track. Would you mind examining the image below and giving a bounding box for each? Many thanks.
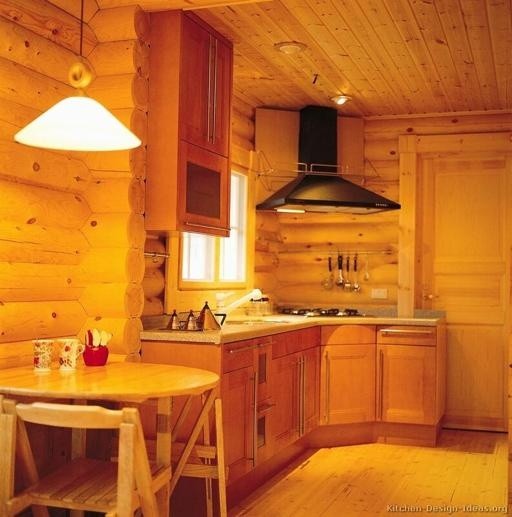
[57,338,88,373]
[30,339,55,374]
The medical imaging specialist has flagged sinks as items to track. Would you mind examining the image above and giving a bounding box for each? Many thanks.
[225,321,285,324]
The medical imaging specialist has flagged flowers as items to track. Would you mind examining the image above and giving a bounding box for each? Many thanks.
[85,327,113,347]
[1,357,228,516]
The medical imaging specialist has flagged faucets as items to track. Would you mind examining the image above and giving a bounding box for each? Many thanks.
[216,288,263,323]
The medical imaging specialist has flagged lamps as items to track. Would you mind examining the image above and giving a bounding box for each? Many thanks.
[12,0,145,154]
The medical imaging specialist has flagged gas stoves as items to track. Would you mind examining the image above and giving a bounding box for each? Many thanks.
[277,308,365,317]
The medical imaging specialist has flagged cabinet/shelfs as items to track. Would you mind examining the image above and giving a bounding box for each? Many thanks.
[222,334,276,487]
[146,9,234,239]
[320,325,437,426]
[273,324,321,459]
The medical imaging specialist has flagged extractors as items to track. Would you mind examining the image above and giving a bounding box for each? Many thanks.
[256,104,402,216]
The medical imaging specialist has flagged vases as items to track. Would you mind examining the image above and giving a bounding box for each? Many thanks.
[83,345,109,366]
